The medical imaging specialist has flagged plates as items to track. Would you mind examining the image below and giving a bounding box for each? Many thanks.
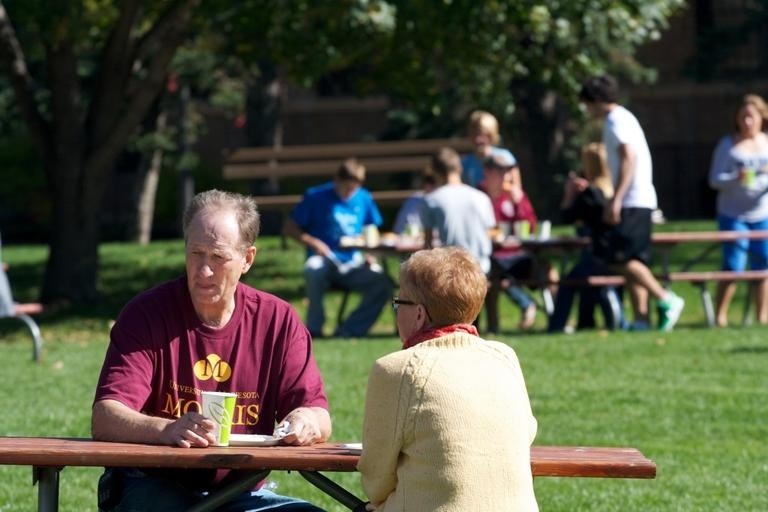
[228,434,281,447]
[344,442,364,456]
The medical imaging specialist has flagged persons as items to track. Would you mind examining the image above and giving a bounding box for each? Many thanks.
[709,95,768,329]
[358,248,539,512]
[544,74,684,336]
[391,110,539,335]
[95,187,332,512]
[286,155,394,337]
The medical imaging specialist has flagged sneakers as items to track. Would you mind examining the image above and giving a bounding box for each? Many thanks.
[657,290,685,332]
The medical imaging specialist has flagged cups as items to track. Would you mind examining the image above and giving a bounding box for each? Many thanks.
[404,215,423,241]
[740,166,757,188]
[514,220,555,242]
[200,390,238,450]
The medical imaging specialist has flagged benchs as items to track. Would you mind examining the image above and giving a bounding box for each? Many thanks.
[222,141,490,249]
[484,271,768,327]
[12,303,43,363]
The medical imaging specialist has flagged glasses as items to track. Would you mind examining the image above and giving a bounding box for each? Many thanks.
[392,296,415,309]
